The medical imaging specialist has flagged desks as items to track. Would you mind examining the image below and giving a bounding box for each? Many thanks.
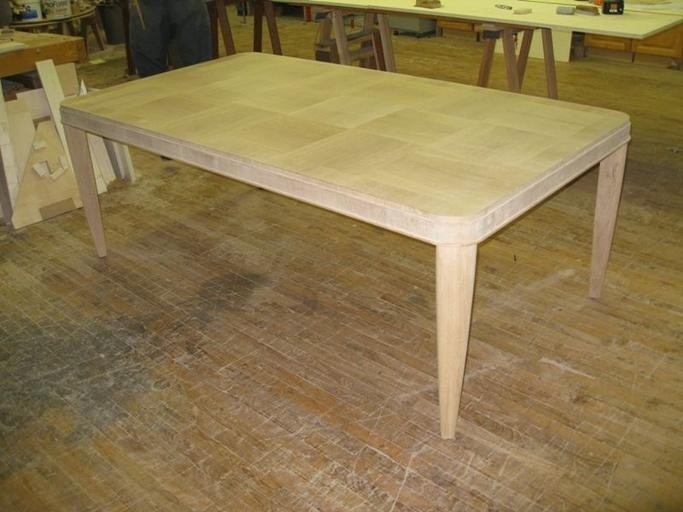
[61,52,632,441]
[4,7,103,51]
[1,29,86,100]
[312,3,681,98]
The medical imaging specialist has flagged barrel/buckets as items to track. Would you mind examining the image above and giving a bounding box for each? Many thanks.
[8,0,43,22]
